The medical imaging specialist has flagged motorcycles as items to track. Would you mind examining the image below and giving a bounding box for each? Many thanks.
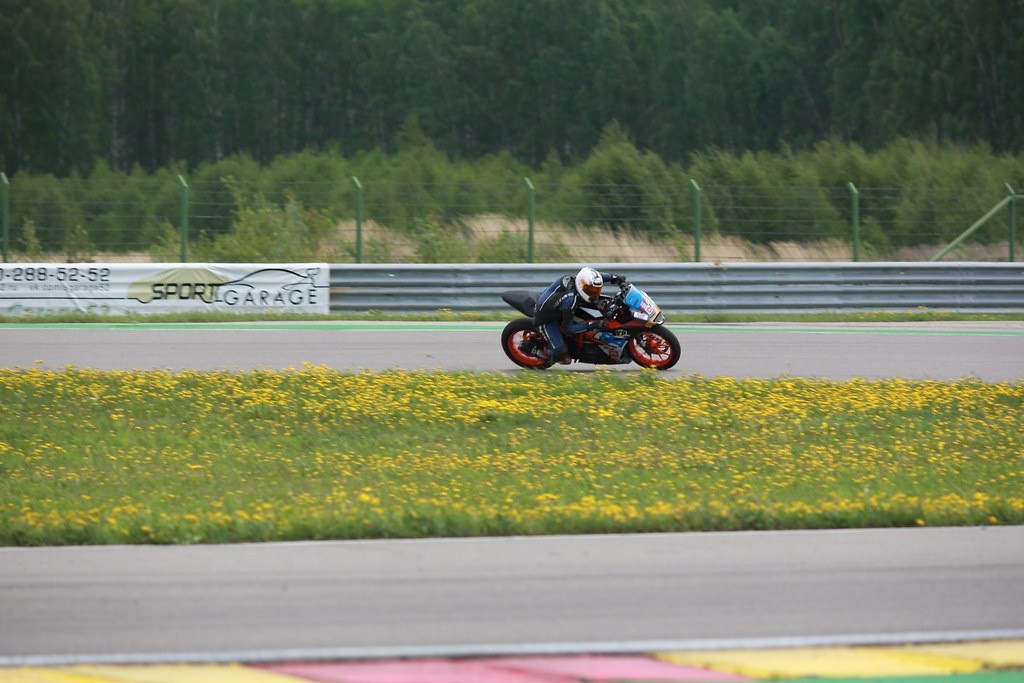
[500,281,682,371]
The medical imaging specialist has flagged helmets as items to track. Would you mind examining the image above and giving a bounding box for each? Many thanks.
[574,267,603,306]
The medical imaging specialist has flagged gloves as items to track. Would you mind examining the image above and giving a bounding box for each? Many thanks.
[610,274,627,284]
[587,320,610,333]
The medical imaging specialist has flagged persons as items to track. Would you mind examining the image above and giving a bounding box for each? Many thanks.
[532,267,626,365]
